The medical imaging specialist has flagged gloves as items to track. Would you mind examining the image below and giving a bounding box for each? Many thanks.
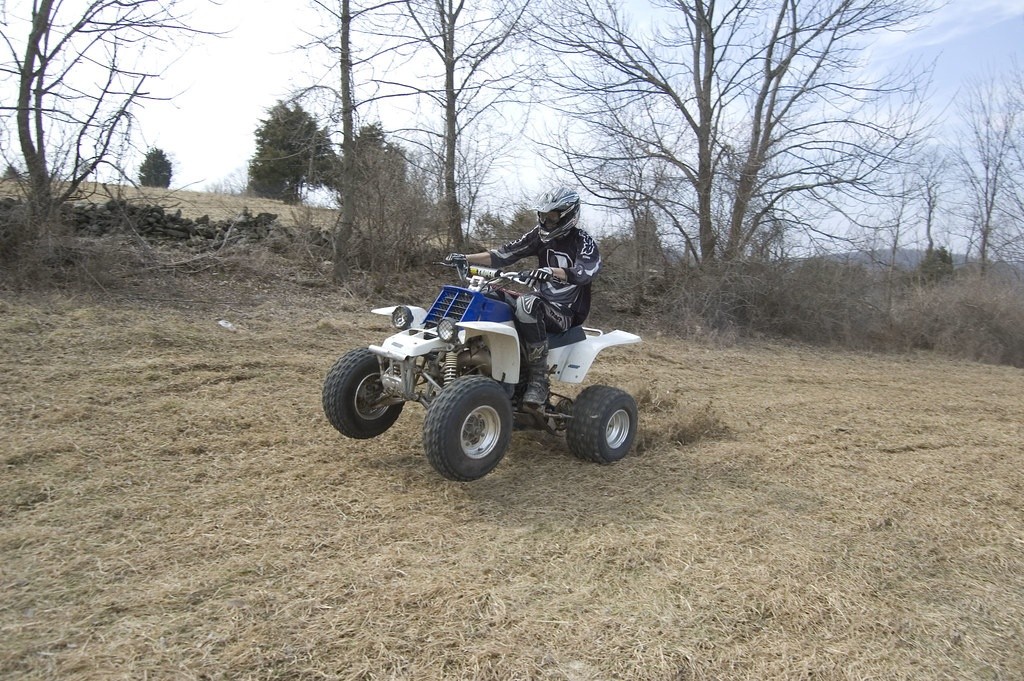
[445,253,466,264]
[529,267,553,283]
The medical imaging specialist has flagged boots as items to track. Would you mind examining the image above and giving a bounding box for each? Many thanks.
[523,336,549,404]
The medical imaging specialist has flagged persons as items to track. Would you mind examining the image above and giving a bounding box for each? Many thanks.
[445,184,602,406]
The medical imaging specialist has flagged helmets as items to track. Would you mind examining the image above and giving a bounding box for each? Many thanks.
[533,187,580,243]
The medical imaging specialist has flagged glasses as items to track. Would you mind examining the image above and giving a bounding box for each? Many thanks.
[540,199,580,224]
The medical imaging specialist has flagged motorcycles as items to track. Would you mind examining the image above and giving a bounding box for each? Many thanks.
[323,252,644,483]
